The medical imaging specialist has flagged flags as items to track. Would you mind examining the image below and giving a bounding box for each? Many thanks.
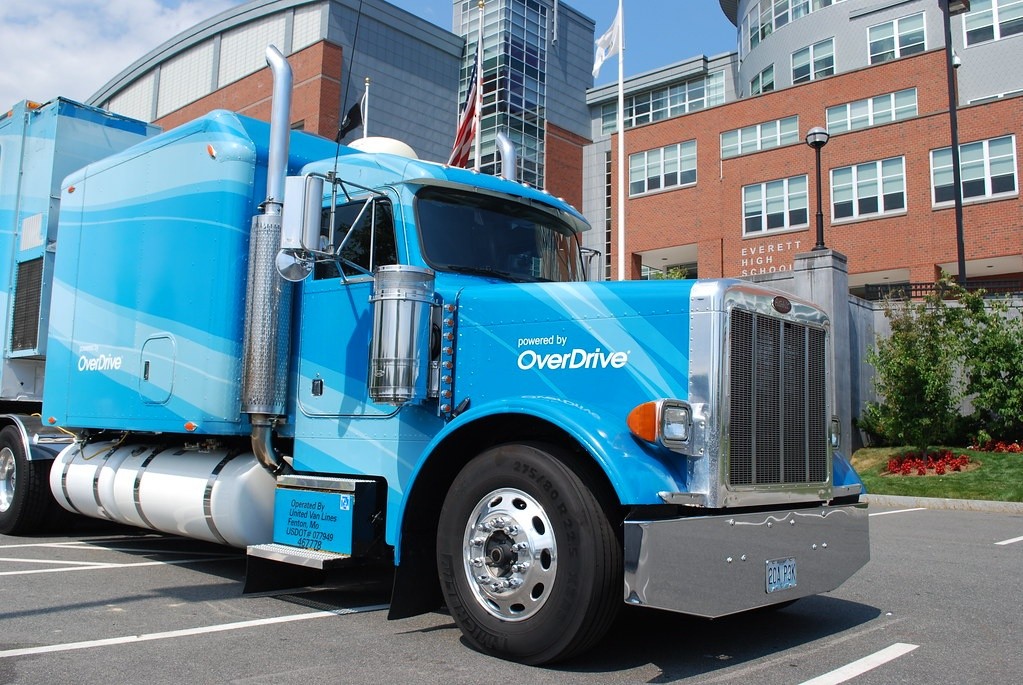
[336,91,363,142]
[448,32,485,168]
[593,4,626,79]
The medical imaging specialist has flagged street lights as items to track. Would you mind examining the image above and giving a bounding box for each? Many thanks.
[803,126,832,250]
[937,1,971,294]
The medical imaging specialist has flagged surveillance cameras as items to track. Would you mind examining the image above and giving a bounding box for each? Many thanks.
[953,56,961,68]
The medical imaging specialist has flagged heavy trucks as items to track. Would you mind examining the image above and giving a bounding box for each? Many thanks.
[0,39,870,665]
